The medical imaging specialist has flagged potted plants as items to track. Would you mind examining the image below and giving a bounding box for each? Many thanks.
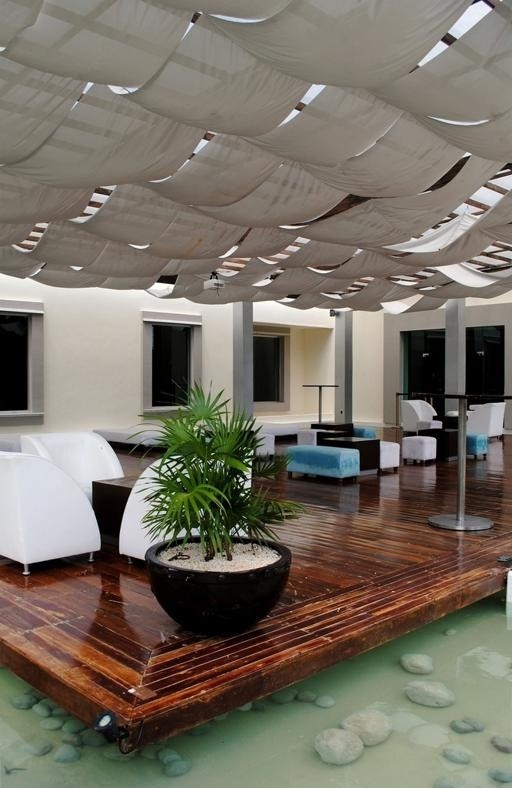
[130,377,306,633]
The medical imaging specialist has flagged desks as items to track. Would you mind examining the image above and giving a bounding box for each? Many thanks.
[91,473,138,535]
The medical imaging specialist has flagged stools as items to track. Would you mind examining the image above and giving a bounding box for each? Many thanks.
[92,394,506,485]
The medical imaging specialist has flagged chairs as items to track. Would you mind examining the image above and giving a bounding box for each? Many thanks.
[19,427,125,498]
[0,447,105,574]
[117,455,252,565]
